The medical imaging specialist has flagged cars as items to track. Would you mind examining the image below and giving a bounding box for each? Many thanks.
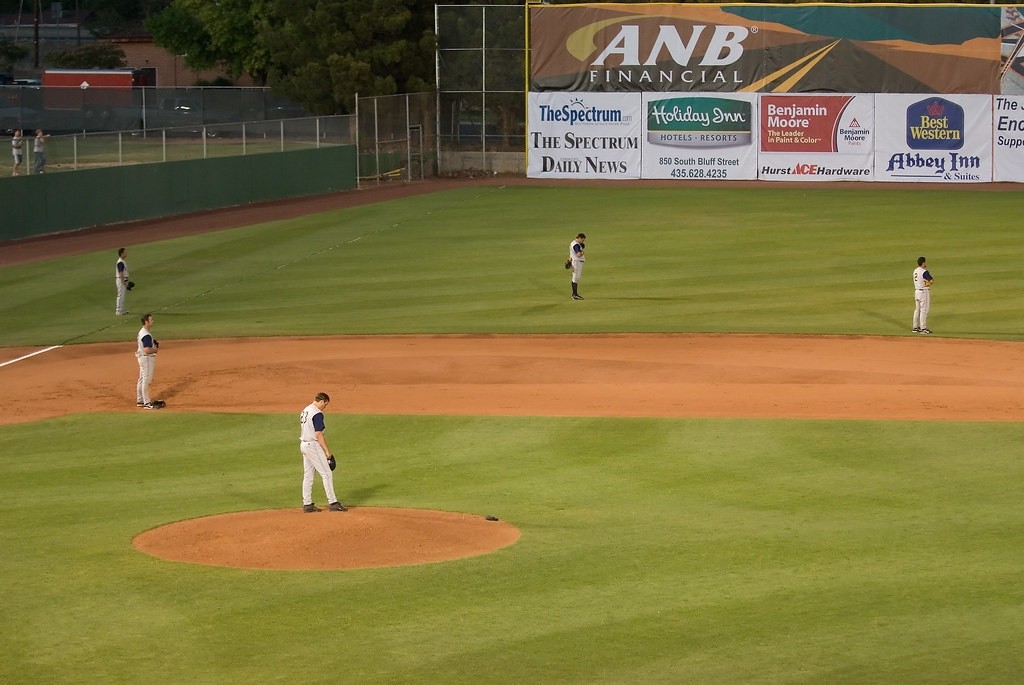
[143,96,240,139]
[0,89,44,137]
[247,100,311,138]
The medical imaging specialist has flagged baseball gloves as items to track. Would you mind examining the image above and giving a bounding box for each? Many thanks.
[126,282,135,291]
[564,259,572,270]
[326,454,337,472]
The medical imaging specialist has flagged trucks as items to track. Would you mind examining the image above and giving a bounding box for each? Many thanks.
[13,69,147,137]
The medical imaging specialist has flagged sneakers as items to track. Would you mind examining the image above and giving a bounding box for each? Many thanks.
[304,503,322,512]
[329,502,346,511]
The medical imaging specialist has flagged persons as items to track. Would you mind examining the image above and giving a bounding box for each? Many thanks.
[32,129,46,175]
[10,129,25,176]
[137,314,159,409]
[913,257,934,334]
[300,392,348,512]
[568,233,586,300]
[116,247,129,316]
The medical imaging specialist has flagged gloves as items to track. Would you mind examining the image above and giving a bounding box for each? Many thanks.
[327,455,336,471]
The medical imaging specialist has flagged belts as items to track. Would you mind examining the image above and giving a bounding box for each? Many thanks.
[580,260,584,262]
[915,289,930,291]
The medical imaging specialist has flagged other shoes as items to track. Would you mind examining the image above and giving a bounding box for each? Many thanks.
[137,403,144,407]
[919,329,932,334]
[912,327,921,332]
[572,294,583,300]
[145,403,153,407]
